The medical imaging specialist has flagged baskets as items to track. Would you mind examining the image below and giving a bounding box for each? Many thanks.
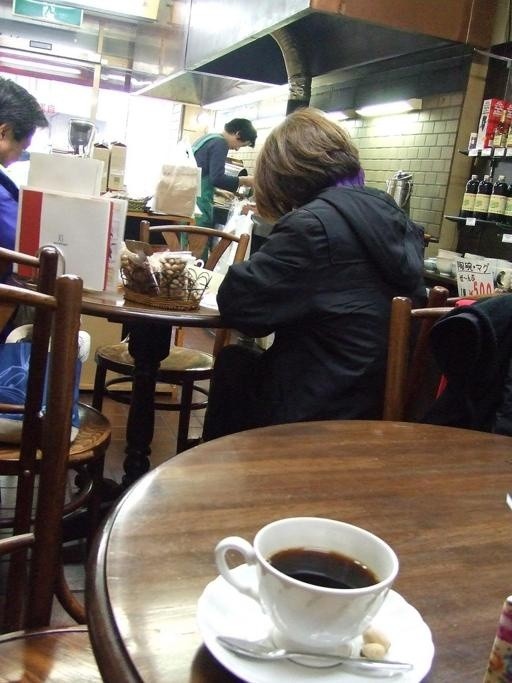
[118,262,212,310]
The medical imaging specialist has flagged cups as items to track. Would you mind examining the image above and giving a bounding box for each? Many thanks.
[496,267,512,292]
[214,515,400,668]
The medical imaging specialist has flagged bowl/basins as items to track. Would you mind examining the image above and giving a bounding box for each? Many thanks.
[422,248,495,284]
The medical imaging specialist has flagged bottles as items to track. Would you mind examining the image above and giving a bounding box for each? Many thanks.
[459,174,512,226]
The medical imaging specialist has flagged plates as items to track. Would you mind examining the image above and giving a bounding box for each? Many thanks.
[196,559,435,683]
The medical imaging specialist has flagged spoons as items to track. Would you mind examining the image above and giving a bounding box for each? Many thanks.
[217,636,413,669]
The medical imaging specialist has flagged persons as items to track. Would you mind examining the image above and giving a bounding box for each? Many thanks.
[198,104,432,442]
[0,73,92,461]
[174,116,255,268]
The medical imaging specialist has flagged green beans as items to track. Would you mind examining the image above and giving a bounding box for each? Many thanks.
[120,247,201,300]
[360,626,392,661]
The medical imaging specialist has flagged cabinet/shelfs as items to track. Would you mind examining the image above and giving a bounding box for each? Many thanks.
[444,147,512,231]
